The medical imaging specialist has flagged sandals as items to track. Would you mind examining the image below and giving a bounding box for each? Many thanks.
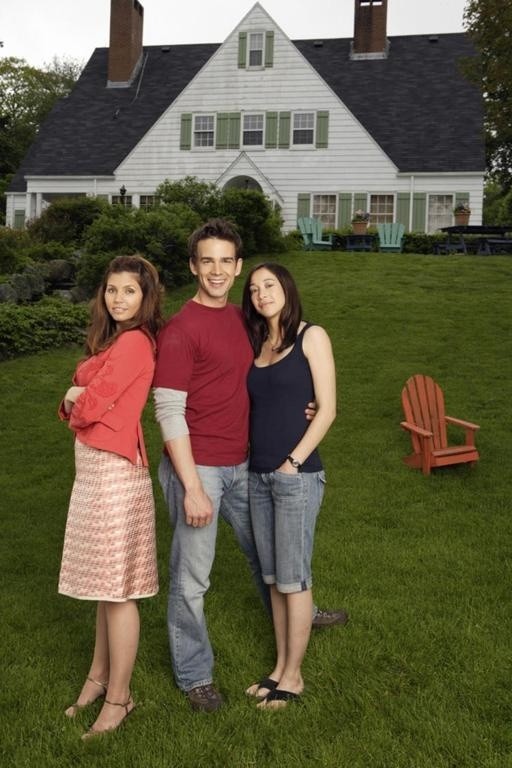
[81,692,135,741]
[65,676,108,718]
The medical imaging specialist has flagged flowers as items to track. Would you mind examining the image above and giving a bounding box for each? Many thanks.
[353,209,370,222]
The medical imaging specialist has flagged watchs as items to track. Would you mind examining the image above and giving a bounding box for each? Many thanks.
[286,453,301,469]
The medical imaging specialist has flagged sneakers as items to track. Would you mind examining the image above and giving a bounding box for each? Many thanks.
[312,608,348,628]
[188,683,221,714]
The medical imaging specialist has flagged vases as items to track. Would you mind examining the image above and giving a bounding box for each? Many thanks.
[351,221,368,234]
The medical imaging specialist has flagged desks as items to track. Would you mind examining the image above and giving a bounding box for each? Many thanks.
[341,234,372,251]
[438,226,511,255]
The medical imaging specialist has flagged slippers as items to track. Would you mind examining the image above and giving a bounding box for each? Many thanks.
[246,676,279,698]
[257,687,300,712]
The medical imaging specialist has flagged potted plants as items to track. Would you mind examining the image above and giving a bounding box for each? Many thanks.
[454,203,471,226]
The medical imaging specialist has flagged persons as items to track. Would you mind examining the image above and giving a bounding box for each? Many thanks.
[148,216,349,713]
[243,265,340,715]
[55,252,164,742]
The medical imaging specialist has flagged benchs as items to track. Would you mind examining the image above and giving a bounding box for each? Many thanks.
[477,238,512,254]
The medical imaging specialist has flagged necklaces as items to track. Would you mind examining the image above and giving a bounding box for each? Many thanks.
[266,333,282,352]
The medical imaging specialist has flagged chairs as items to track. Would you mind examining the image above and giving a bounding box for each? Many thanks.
[298,216,333,251]
[400,373,481,477]
[377,222,405,253]
